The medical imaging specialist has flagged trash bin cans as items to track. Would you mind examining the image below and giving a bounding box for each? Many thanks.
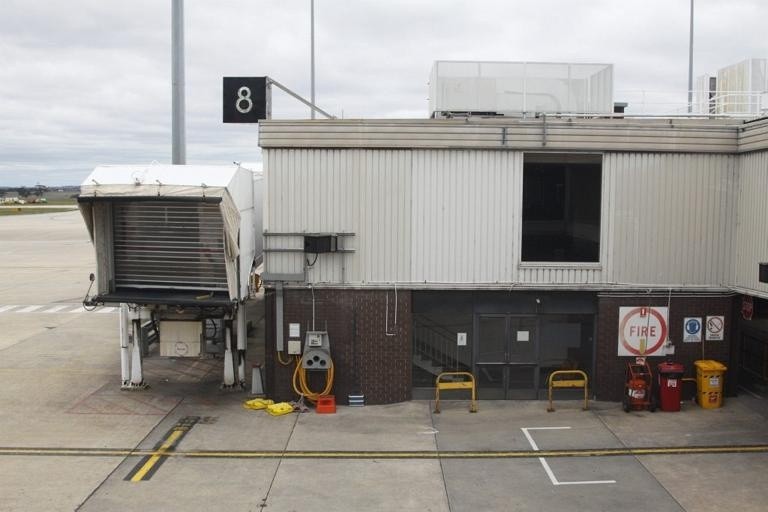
[694,360,727,408]
[655,361,685,411]
